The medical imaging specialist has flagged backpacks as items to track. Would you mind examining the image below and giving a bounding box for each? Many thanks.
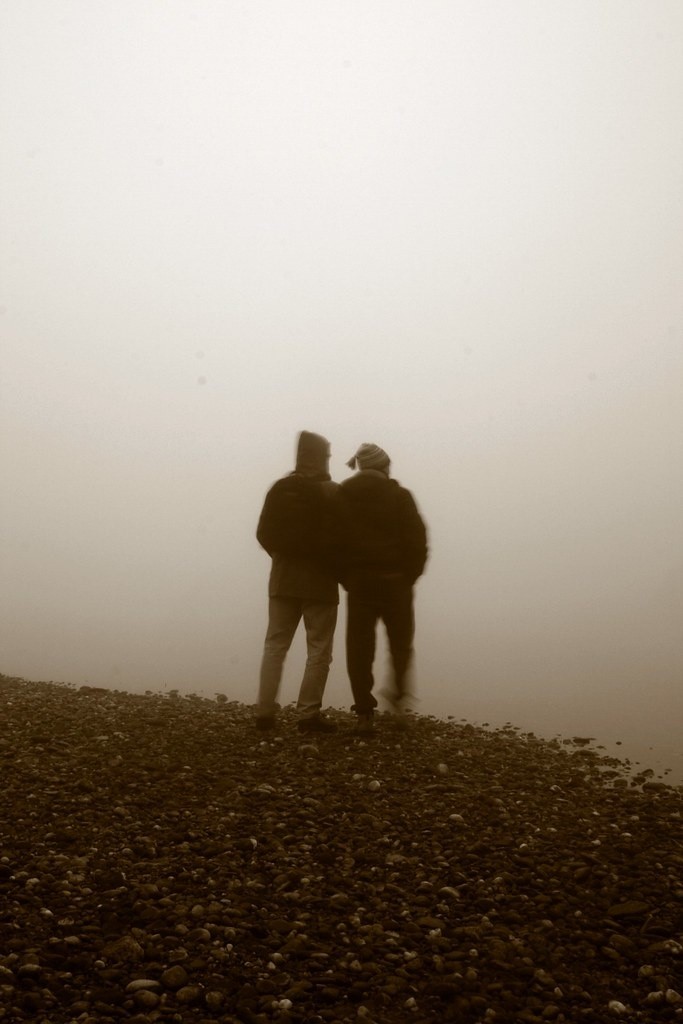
[264,474,327,551]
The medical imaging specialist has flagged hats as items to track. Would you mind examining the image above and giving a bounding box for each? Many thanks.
[347,443,390,470]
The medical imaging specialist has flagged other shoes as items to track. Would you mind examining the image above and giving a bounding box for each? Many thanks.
[299,719,338,733]
[352,714,377,736]
[256,718,274,729]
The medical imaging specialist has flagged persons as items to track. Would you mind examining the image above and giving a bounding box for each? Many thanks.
[327,442,428,732]
[256,429,341,733]
